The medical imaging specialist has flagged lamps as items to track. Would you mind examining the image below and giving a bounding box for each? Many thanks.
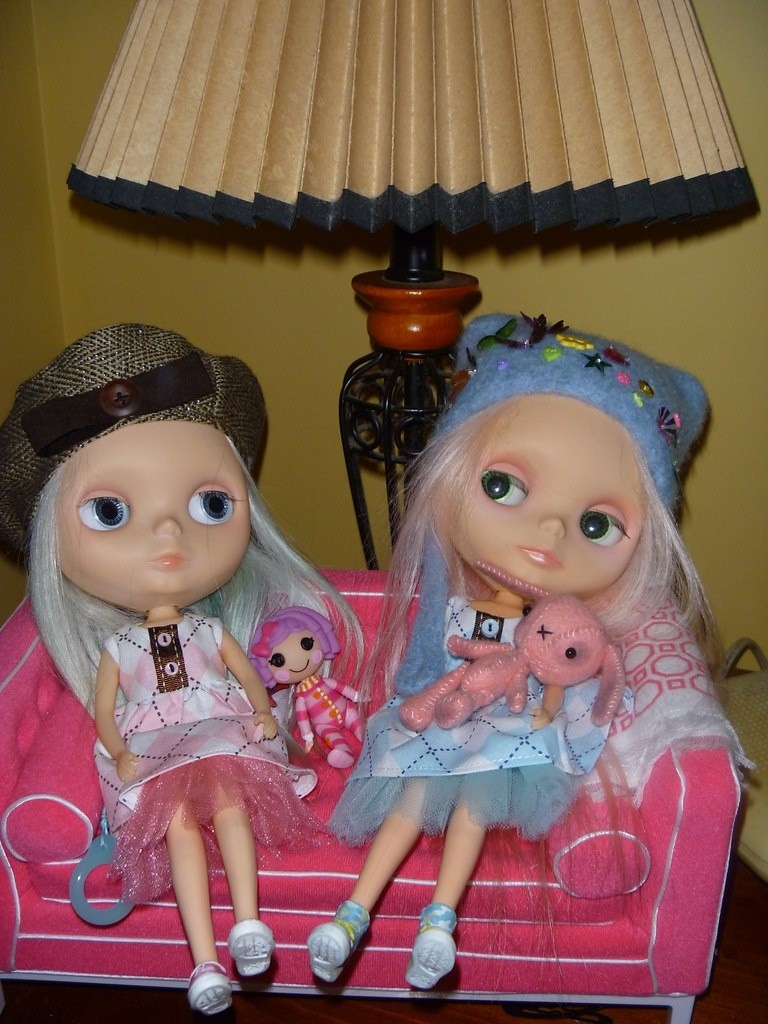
[64,0,762,577]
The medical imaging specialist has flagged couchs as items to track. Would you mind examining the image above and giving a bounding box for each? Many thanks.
[1,571,756,1024]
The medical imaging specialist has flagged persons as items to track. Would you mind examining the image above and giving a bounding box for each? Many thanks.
[307,314,711,989]
[250,606,372,768]
[0,322,363,1015]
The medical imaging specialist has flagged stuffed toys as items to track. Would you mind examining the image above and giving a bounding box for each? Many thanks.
[397,560,625,733]
